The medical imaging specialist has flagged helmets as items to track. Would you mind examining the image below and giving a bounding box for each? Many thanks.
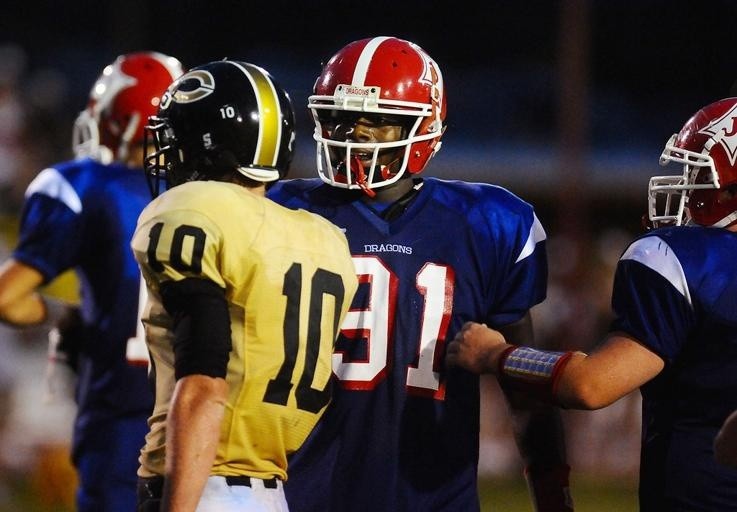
[307,36,446,190]
[144,61,297,200]
[648,97,737,229]
[73,50,186,166]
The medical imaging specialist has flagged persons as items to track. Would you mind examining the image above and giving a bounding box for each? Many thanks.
[434,95,735,511]
[124,55,358,511]
[0,47,188,509]
[256,33,577,512]
[0,57,79,511]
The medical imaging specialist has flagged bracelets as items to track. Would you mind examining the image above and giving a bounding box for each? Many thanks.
[503,339,581,392]
